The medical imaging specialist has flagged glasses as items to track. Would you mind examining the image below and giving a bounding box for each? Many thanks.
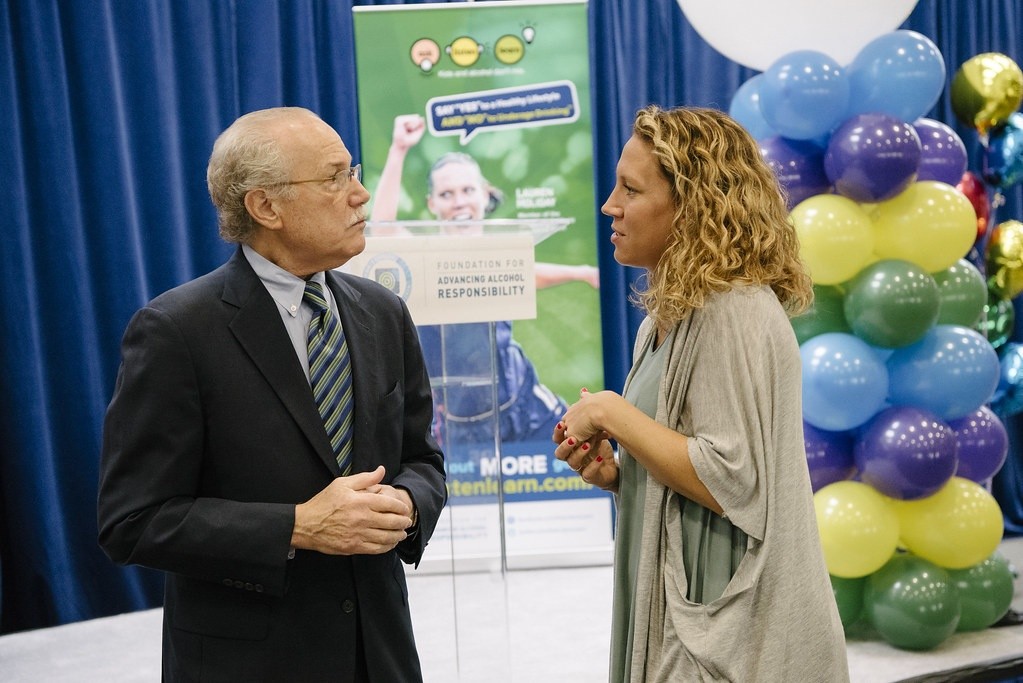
[257,164,362,190]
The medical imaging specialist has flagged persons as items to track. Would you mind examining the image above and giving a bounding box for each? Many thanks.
[97,107,450,682]
[371,111,598,450]
[555,103,848,682]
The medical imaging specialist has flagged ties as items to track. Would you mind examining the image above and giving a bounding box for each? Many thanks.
[302,281,354,477]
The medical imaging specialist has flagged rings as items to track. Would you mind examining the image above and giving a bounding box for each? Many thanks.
[568,464,583,472]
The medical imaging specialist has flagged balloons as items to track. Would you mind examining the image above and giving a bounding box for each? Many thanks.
[649,0,1021,653]
